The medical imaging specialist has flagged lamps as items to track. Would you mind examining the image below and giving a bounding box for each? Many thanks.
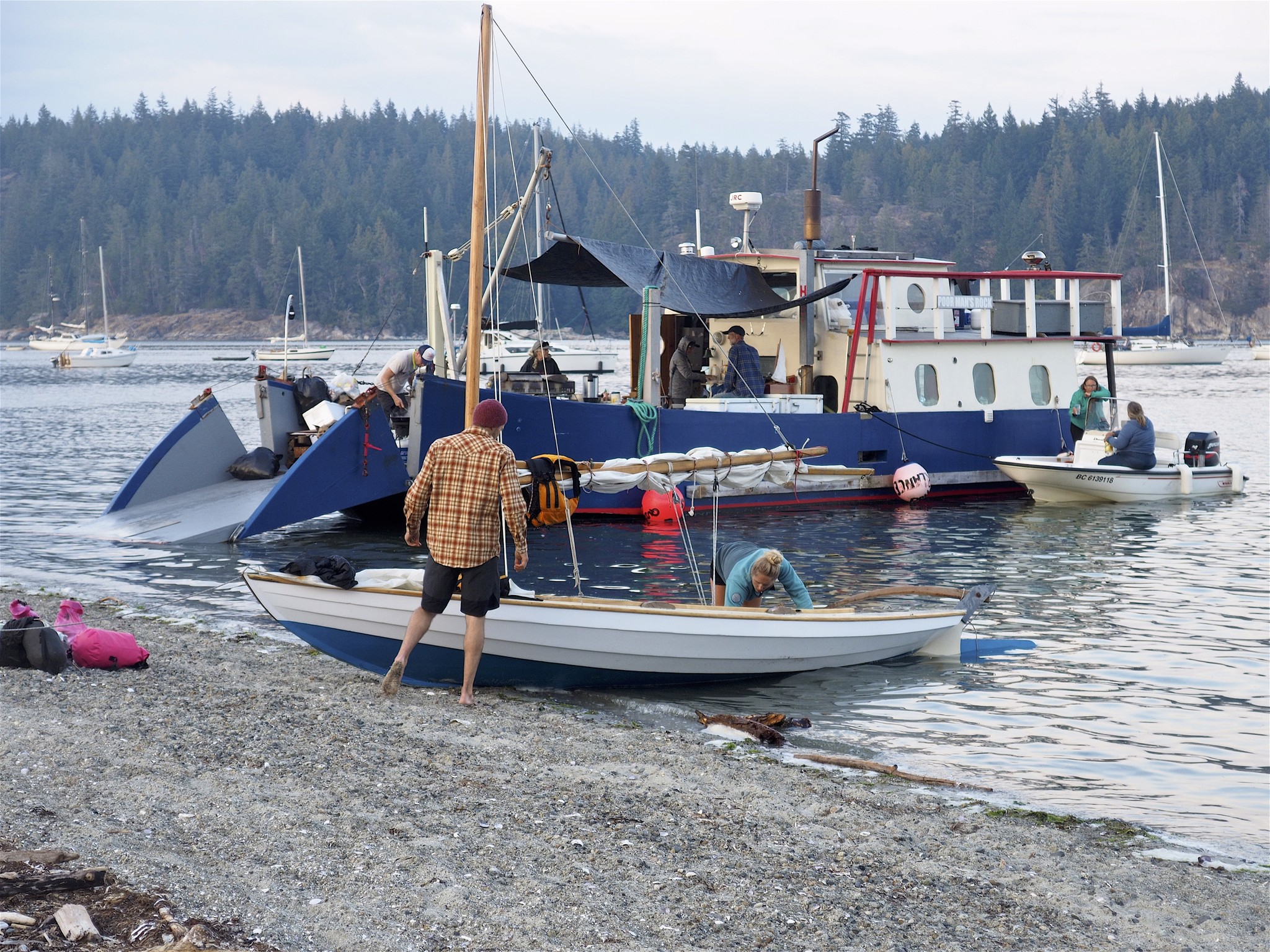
[702,345,716,358]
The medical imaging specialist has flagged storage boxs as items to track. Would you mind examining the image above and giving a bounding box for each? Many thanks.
[766,384,794,394]
[765,393,824,414]
[684,398,780,414]
[302,400,346,430]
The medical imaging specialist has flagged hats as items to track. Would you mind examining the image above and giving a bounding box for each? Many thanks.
[472,398,507,428]
[419,345,436,366]
[689,341,700,347]
[722,325,745,336]
[533,340,552,352]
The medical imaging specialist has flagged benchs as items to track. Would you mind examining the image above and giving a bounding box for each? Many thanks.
[991,299,1109,337]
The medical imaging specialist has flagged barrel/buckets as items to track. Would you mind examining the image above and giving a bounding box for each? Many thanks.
[583,373,599,403]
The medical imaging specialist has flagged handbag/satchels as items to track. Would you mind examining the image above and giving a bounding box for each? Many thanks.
[0,597,150,671]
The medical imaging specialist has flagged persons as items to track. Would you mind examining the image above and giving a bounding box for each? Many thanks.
[1068,376,1111,447]
[372,345,435,422]
[1098,401,1157,470]
[708,541,814,610]
[518,339,562,375]
[382,399,529,705]
[712,325,765,398]
[668,334,716,410]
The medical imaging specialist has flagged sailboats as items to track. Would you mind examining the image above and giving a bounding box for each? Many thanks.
[252,246,337,363]
[1073,130,1270,365]
[212,1,967,693]
[27,216,137,370]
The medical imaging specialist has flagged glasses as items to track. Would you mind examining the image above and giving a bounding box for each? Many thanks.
[536,341,550,349]
[689,346,694,350]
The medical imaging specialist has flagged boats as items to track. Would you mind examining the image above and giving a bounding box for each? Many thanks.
[211,356,249,361]
[993,398,1252,508]
[112,130,1124,561]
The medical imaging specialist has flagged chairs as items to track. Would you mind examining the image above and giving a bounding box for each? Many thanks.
[1153,431,1181,466]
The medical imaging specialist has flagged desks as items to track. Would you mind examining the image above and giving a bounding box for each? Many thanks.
[702,383,724,398]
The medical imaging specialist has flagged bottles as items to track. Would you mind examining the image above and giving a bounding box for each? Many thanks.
[711,364,721,380]
[611,392,621,404]
